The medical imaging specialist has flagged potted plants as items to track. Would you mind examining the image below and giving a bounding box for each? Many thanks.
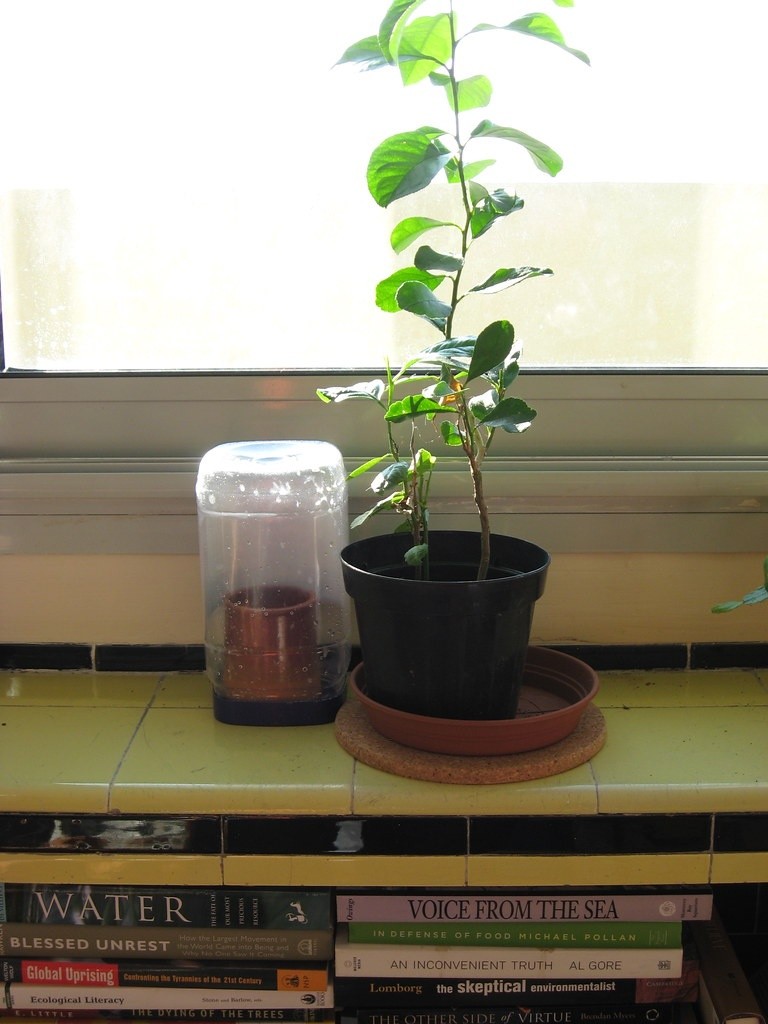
[314,0,594,724]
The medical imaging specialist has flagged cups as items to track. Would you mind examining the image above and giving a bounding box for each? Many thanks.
[194,441,353,726]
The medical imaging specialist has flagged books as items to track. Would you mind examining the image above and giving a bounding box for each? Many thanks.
[1,883,336,1024]
[332,887,768,1024]
